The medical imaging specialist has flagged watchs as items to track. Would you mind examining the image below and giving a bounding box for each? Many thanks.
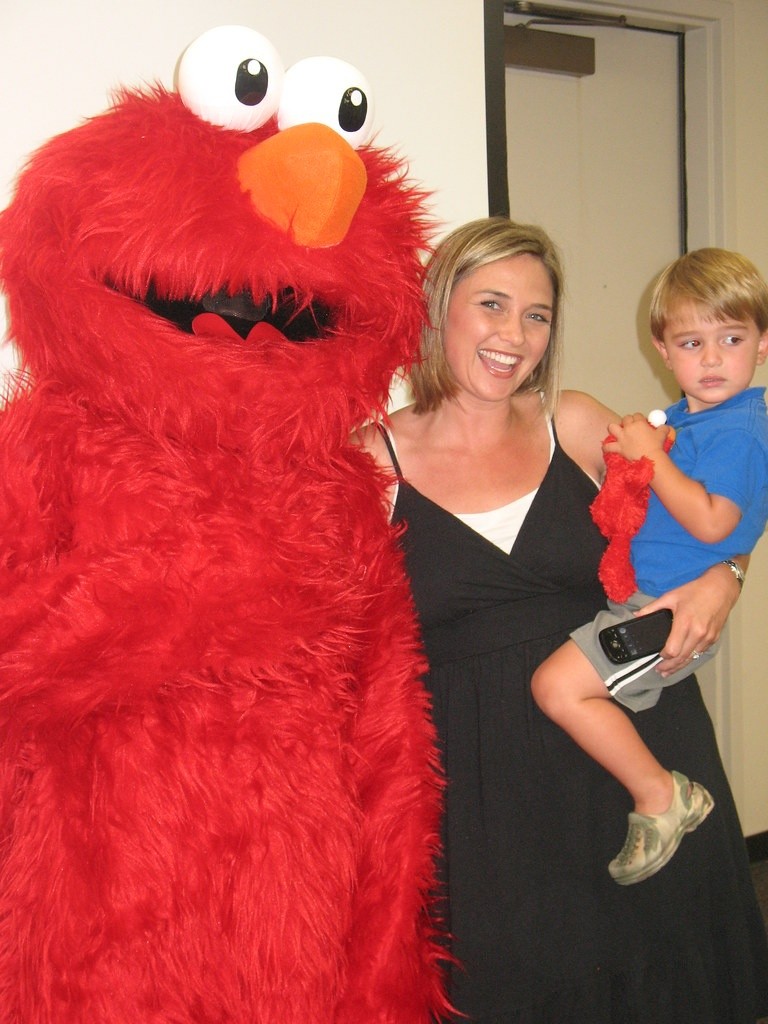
[722,560,745,591]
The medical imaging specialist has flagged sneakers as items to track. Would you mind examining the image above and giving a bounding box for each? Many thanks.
[607,770,716,885]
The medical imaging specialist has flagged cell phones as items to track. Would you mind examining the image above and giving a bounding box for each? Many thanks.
[599,609,673,665]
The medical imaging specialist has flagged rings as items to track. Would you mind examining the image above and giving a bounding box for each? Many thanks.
[689,650,703,660]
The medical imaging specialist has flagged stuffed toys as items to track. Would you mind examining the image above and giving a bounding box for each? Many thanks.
[0,23,444,1024]
[589,409,676,603]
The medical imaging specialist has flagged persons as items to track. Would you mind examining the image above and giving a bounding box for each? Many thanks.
[347,216,768,1024]
[530,247,768,886]
